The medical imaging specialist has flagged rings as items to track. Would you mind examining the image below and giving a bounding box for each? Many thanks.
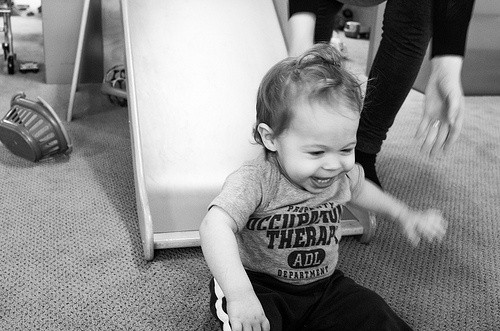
[429,120,439,129]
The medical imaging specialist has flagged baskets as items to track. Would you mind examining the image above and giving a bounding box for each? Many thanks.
[0,93,71,162]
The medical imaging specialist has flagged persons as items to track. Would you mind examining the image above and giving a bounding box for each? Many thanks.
[287,0,476,193]
[198,59,449,331]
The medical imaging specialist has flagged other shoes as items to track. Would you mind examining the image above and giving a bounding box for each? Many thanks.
[355,147,382,190]
